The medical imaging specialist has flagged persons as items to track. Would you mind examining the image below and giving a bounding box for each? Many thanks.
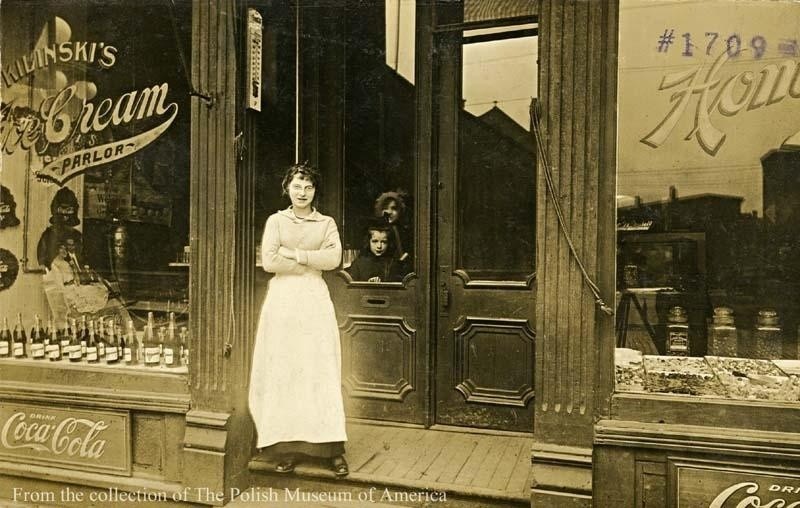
[370,187,416,268]
[348,219,405,284]
[66,234,92,282]
[49,242,74,285]
[248,159,352,480]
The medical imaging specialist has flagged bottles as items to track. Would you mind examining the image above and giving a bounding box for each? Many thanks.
[130,193,164,221]
[706,306,740,358]
[756,310,782,358]
[176,245,189,263]
[664,304,691,355]
[0,311,188,369]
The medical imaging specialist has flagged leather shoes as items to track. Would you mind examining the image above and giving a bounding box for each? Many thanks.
[331,458,350,477]
[274,456,297,474]
